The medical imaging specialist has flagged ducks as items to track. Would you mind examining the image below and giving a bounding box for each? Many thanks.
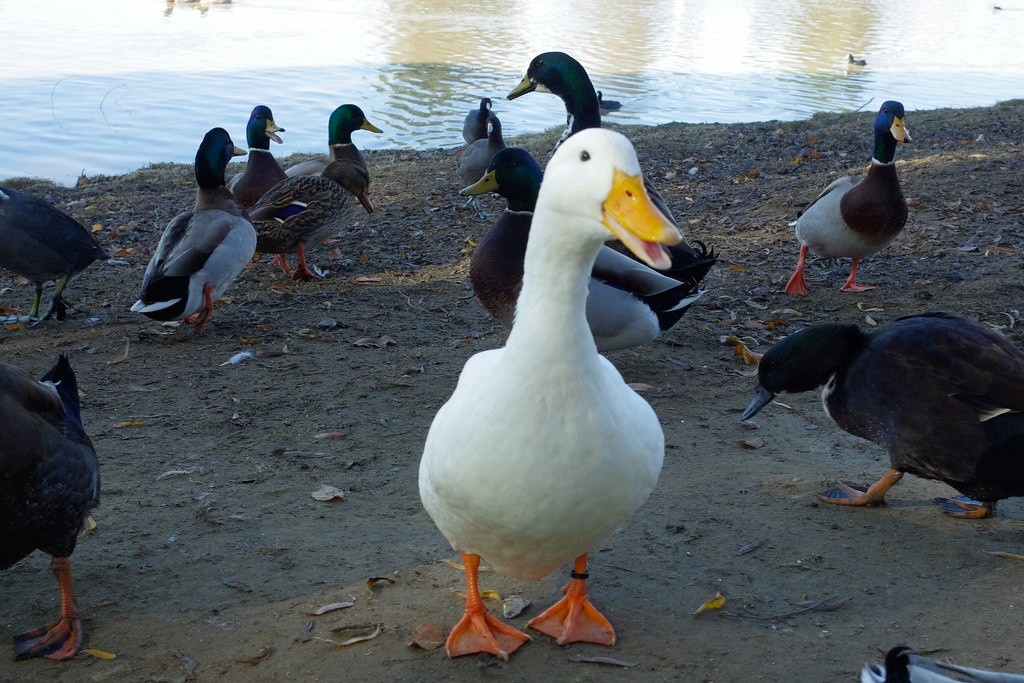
[0,49,1024,663]
